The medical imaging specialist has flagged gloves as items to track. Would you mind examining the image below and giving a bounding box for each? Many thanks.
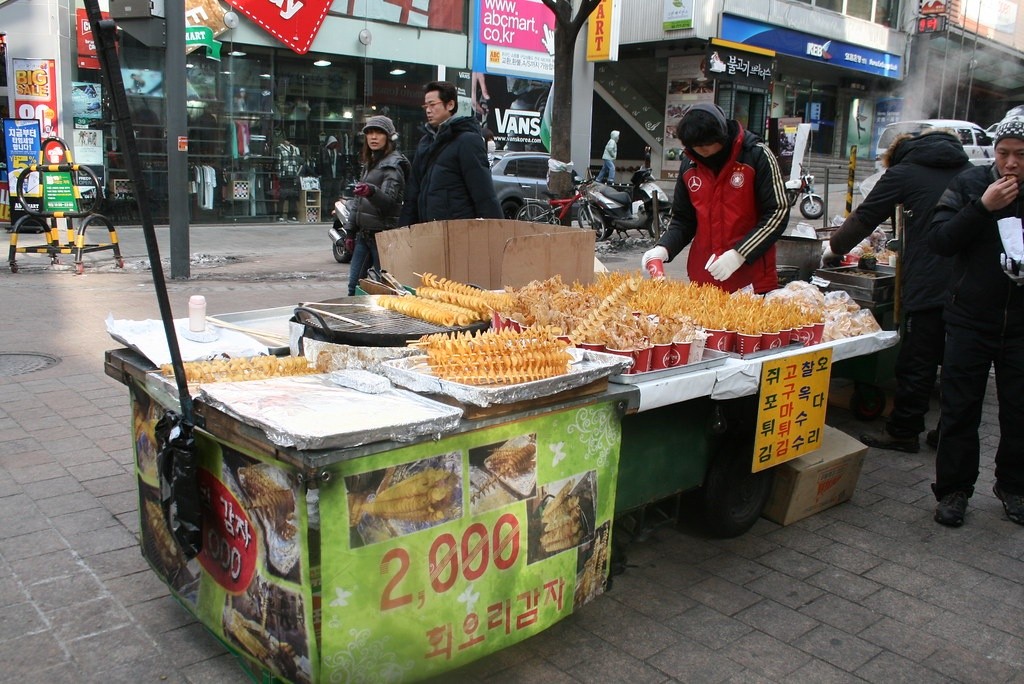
[641,246,668,272]
[708,248,748,281]
[1000,252,1024,287]
[344,235,356,254]
[821,246,843,268]
[352,184,369,196]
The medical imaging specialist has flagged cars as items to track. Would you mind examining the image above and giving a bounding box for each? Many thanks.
[982,122,999,138]
[490,148,587,223]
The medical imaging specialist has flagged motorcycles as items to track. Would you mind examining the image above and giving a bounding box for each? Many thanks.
[327,182,358,264]
[785,163,826,220]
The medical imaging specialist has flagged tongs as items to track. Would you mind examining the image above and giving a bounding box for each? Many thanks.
[297,301,370,328]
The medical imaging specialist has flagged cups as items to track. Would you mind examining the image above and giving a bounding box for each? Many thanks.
[645,256,666,283]
[483,289,826,374]
[188,295,207,332]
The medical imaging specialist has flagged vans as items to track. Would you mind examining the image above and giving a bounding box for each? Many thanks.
[873,119,996,167]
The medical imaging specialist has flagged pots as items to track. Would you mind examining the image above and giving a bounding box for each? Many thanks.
[293,283,492,348]
[774,235,824,289]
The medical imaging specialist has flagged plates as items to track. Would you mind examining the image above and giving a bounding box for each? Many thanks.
[484,437,536,499]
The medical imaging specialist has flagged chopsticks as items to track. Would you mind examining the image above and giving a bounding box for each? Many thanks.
[205,316,290,347]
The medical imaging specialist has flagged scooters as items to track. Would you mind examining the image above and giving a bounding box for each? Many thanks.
[583,145,673,240]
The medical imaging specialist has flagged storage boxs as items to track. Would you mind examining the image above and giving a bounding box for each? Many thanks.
[761,421,870,526]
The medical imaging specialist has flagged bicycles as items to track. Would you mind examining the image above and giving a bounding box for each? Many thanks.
[514,175,606,242]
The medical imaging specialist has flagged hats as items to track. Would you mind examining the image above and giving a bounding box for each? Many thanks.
[994,105,1024,151]
[362,116,396,137]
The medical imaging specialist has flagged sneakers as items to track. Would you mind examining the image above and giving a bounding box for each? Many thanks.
[860,429,921,452]
[992,481,1024,525]
[930,482,968,525]
[926,430,939,448]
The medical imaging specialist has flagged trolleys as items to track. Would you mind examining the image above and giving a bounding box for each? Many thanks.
[775,231,948,423]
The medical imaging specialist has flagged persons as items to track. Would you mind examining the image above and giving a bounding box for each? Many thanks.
[398,80,503,227]
[320,137,342,219]
[822,126,973,451]
[596,130,620,183]
[931,104,1024,528]
[642,103,790,296]
[276,141,301,224]
[347,116,412,295]
[471,74,550,151]
[482,129,495,150]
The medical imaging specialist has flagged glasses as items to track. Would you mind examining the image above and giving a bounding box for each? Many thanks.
[421,99,451,113]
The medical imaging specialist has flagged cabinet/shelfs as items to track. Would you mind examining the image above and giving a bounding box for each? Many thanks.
[297,190,321,222]
